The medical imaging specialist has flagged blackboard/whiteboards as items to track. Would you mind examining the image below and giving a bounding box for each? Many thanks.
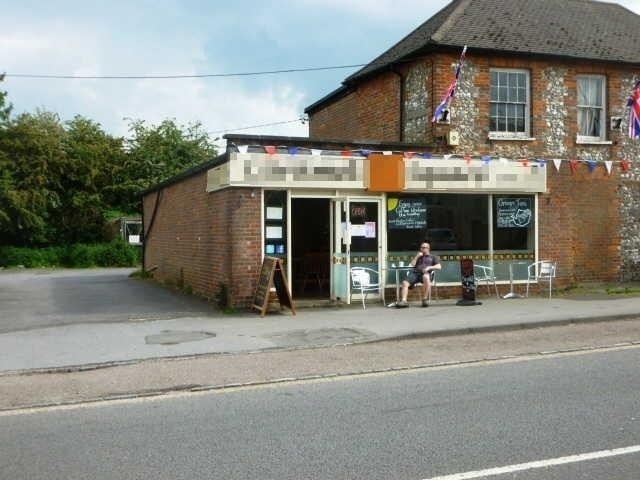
[497,198,532,227]
[253,256,275,310]
[386,199,427,231]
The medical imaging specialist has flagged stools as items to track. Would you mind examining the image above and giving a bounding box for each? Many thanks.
[305,271,322,299]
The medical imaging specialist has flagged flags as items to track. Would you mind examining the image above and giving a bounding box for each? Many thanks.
[624,80,640,141]
[431,44,469,122]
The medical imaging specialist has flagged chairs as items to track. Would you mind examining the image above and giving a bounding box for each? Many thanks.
[526,259,558,300]
[474,266,500,299]
[349,266,440,311]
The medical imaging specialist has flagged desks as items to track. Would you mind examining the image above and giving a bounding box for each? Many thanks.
[292,257,305,297]
[495,260,528,299]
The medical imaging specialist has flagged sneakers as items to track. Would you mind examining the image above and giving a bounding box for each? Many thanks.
[422,299,429,307]
[396,301,409,307]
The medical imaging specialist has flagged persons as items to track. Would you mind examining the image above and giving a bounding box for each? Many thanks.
[395,242,441,309]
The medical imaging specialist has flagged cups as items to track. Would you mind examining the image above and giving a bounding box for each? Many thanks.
[399,262,404,267]
